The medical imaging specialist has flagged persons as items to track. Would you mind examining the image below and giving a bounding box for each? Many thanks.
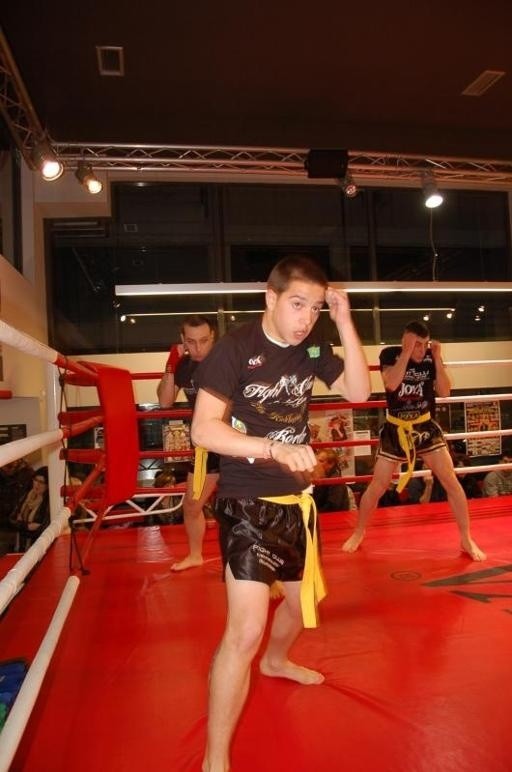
[0,435,35,556]
[362,451,512,507]
[7,466,51,553]
[310,446,351,512]
[155,312,283,600]
[189,253,375,770]
[142,474,183,525]
[340,319,489,562]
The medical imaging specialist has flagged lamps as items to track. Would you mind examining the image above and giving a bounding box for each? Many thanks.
[422,172,443,209]
[337,171,358,197]
[74,162,102,195]
[30,144,64,182]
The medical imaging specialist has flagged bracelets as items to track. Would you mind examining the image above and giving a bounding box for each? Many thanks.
[263,435,270,460]
[268,440,276,462]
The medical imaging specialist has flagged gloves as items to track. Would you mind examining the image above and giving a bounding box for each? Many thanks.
[165,344,183,374]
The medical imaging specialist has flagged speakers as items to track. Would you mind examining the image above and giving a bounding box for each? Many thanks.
[308,147,348,179]
